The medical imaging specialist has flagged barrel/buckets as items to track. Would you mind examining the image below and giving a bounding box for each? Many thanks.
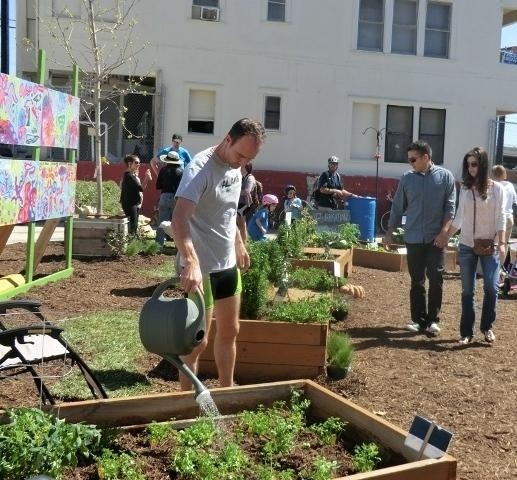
[343,194,377,245]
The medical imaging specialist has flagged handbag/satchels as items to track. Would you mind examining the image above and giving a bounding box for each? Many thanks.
[473,238,495,256]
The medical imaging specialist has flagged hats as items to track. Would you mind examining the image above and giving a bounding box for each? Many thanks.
[492,164,507,180]
[160,150,184,165]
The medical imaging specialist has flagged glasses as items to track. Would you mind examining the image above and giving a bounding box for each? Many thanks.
[466,161,477,169]
[407,155,422,163]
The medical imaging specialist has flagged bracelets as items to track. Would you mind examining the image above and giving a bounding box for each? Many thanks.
[497,241,506,244]
[350,193,353,197]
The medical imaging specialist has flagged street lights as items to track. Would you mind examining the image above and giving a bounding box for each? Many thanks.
[359,126,395,199]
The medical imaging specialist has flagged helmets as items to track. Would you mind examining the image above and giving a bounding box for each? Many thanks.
[283,185,295,195]
[328,156,338,163]
[261,193,279,206]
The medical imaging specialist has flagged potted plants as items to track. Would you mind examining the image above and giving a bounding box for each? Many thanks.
[324,330,359,380]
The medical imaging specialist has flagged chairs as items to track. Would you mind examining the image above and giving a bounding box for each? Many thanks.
[0,299,108,406]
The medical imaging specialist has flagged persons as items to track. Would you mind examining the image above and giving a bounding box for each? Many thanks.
[279,184,303,225]
[236,161,256,244]
[170,117,268,391]
[384,140,456,336]
[155,150,183,247]
[118,153,153,236]
[247,193,279,241]
[317,156,357,210]
[434,146,507,345]
[149,134,192,177]
[491,164,516,292]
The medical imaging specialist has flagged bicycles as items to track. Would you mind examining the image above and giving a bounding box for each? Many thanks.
[379,191,394,234]
[277,192,356,228]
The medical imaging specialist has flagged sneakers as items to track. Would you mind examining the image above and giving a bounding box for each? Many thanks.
[406,322,440,334]
[458,328,495,346]
